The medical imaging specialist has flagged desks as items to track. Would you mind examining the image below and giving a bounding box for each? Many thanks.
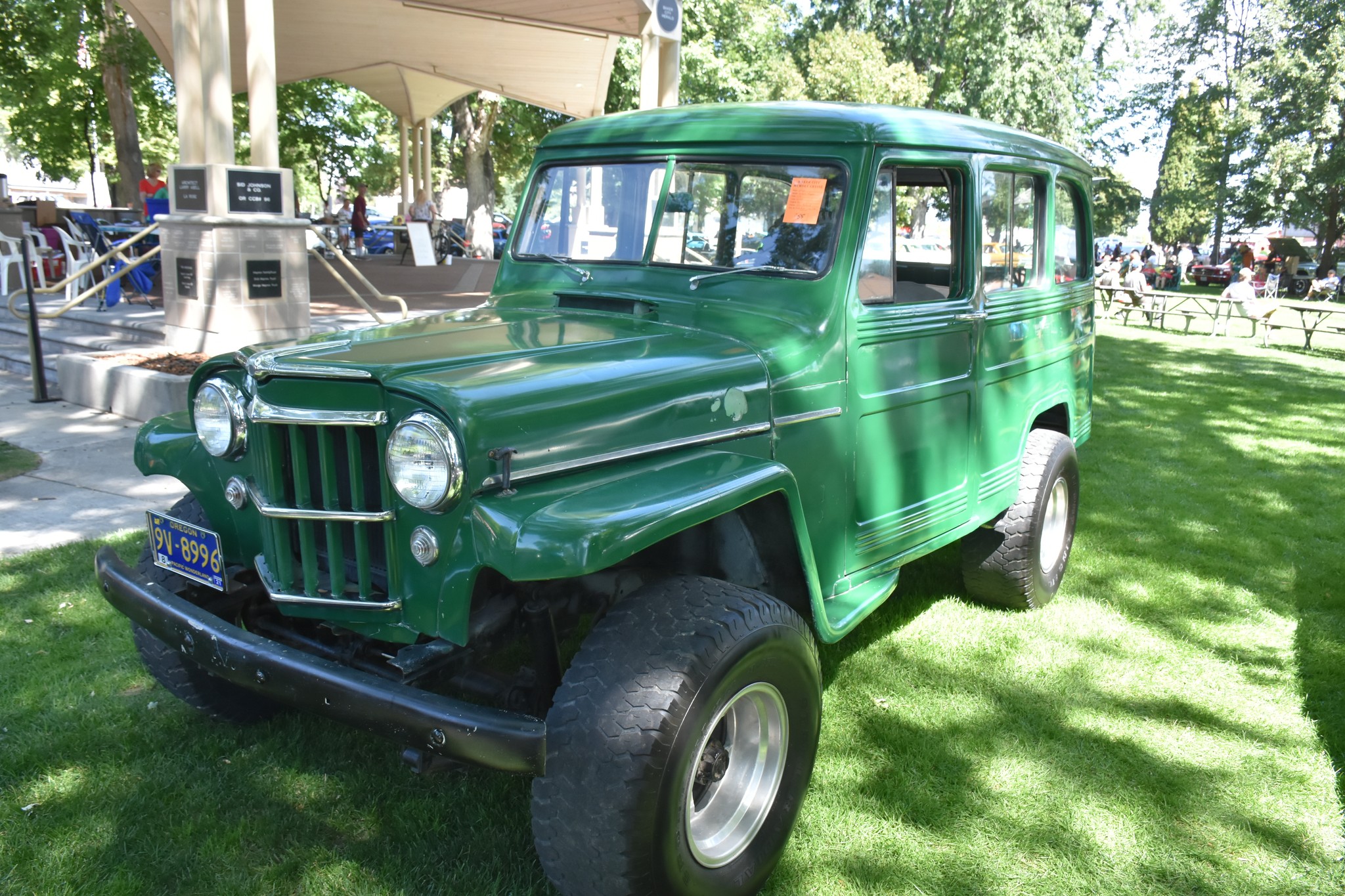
[315,224,352,257]
[370,225,413,265]
[1279,303,1345,350]
[101,226,159,296]
[1142,290,1241,335]
[1094,284,1134,320]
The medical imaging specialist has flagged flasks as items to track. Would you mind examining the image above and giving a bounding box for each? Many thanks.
[0,173,8,199]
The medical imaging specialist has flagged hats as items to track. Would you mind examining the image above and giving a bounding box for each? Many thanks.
[1132,260,1144,267]
[1239,268,1256,276]
[359,184,368,188]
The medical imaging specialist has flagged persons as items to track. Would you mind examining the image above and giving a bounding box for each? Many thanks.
[1094,242,1101,260]
[1221,267,1278,339]
[352,183,372,261]
[1102,245,1113,259]
[337,198,353,254]
[1141,261,1156,290]
[409,189,437,233]
[1095,262,1132,319]
[1124,259,1163,322]
[139,163,169,227]
[1302,269,1340,301]
[1262,243,1275,275]
[1230,244,1255,284]
[1155,261,1178,290]
[1112,242,1123,259]
[1141,245,1157,264]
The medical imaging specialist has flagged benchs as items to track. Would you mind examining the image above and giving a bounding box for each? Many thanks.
[1095,299,1345,336]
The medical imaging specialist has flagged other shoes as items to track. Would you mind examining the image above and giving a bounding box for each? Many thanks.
[353,255,371,262]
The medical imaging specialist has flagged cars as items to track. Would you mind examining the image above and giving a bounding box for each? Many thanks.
[305,204,559,260]
[858,238,1345,297]
[92,98,1095,896]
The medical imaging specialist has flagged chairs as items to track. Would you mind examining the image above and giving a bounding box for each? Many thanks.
[1252,272,1281,298]
[1161,265,1182,289]
[0,211,161,311]
[1312,276,1342,303]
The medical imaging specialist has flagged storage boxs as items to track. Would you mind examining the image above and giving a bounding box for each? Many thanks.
[36,200,56,226]
[32,253,63,279]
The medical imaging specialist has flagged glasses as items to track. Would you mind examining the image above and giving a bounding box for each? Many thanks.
[156,170,161,173]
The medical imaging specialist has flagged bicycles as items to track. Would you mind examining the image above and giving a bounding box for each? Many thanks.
[400,211,451,265]
